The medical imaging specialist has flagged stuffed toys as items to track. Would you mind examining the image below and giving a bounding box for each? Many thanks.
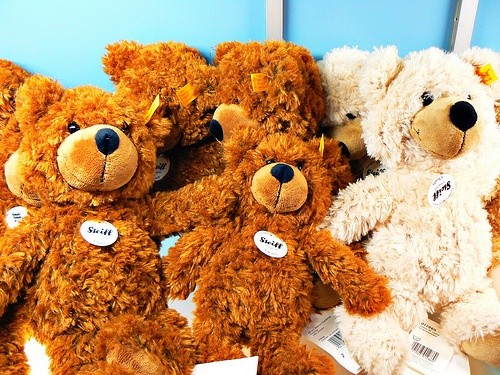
[310,47,390,175]
[322,43,500,374]
[101,37,228,189]
[0,58,44,375]
[211,40,357,311]
[157,124,388,374]
[0,73,237,375]
